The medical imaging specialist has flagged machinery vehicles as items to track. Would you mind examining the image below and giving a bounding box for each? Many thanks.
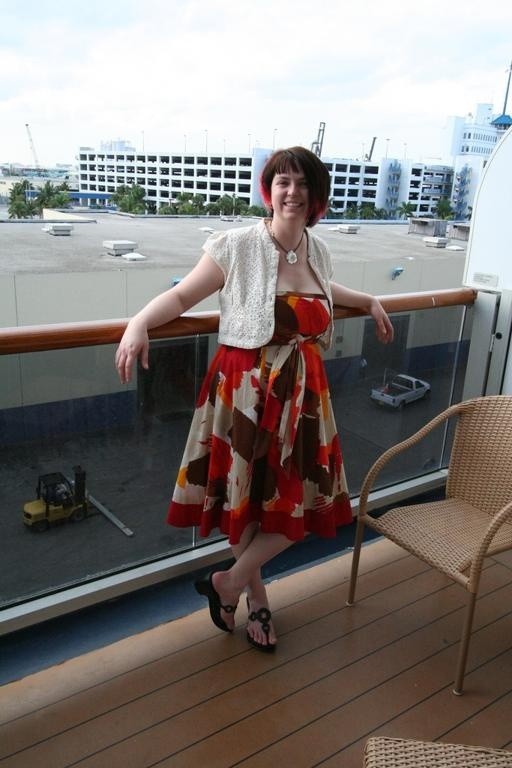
[23,464,109,532]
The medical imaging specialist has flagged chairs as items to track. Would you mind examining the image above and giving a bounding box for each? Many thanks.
[362,734,512,767]
[345,395,512,700]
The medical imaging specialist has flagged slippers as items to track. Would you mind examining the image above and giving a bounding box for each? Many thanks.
[193,570,238,635]
[246,594,278,655]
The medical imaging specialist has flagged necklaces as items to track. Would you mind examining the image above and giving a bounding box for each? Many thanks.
[266,218,306,265]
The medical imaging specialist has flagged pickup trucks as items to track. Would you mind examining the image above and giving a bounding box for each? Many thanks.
[370,371,433,409]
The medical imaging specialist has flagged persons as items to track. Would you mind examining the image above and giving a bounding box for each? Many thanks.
[114,147,395,653]
